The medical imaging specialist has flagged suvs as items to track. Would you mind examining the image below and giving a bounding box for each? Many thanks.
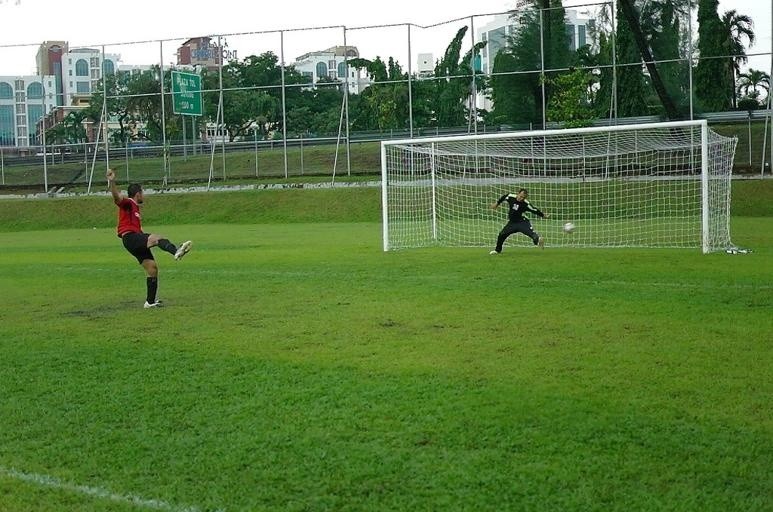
[191,141,212,153]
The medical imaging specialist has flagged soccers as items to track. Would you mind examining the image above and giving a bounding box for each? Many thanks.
[564,223,574,232]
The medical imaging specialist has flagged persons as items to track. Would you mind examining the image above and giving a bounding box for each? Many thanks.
[490,189,550,254]
[105,168,192,308]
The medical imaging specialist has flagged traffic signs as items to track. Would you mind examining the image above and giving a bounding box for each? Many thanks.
[170,71,202,118]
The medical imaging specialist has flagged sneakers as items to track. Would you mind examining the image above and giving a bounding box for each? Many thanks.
[537,236,545,251]
[489,250,499,255]
[173,240,193,263]
[143,298,163,310]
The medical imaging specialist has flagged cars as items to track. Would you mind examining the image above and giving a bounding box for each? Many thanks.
[32,144,125,153]
